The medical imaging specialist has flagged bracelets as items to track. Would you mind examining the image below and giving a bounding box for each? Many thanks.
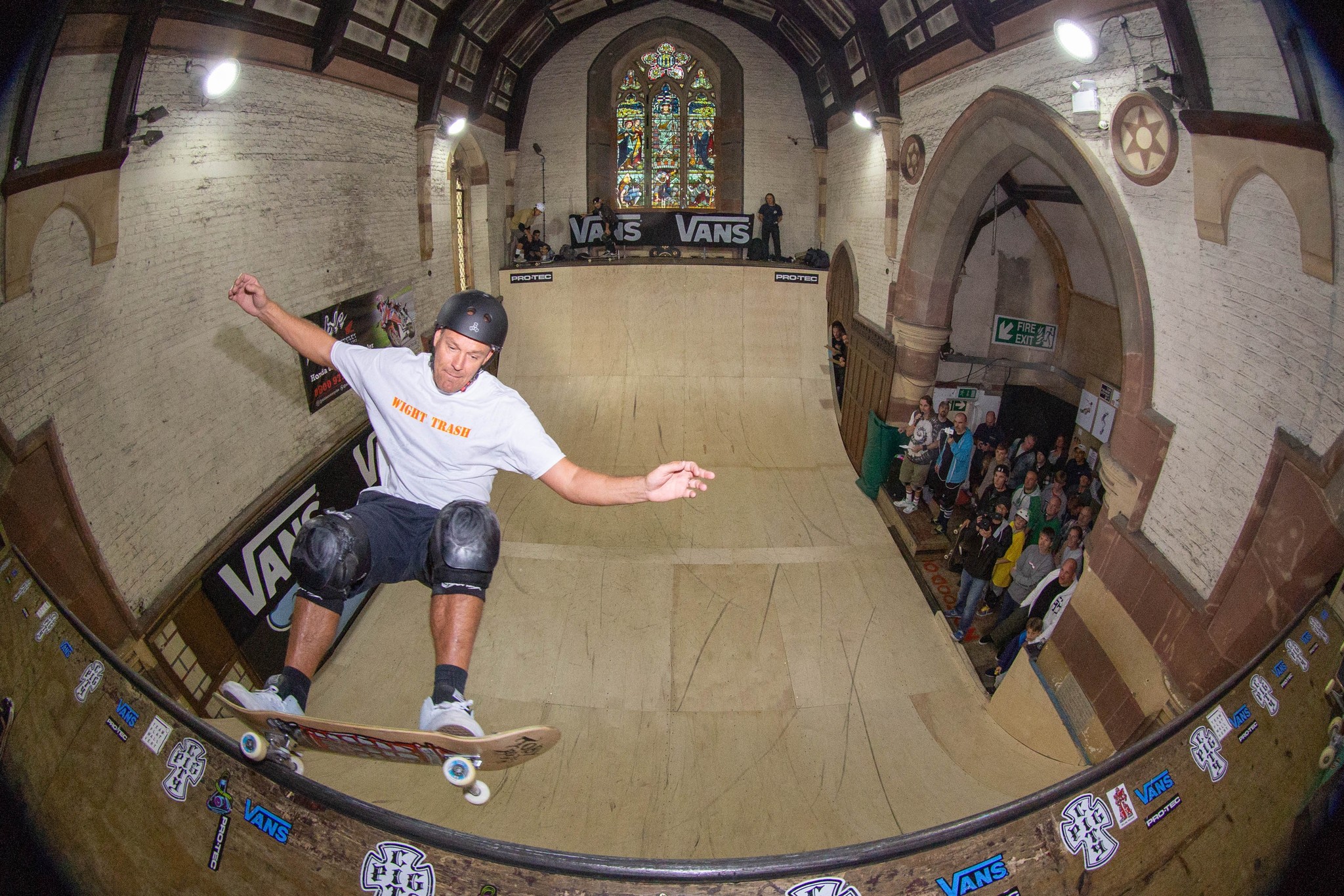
[922,444,927,451]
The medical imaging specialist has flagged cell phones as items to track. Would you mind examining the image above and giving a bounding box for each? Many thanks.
[944,428,954,435]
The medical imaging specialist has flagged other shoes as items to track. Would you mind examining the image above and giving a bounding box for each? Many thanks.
[995,652,1001,660]
[985,686,996,695]
[979,635,991,645]
[986,668,996,677]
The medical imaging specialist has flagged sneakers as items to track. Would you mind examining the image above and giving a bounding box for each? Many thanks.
[416,690,482,739]
[221,679,307,717]
[513,256,526,262]
[929,516,942,524]
[601,252,617,258]
[931,525,946,534]
[951,629,965,641]
[944,609,961,617]
[540,258,552,263]
[893,499,909,507]
[903,502,918,513]
[976,605,992,615]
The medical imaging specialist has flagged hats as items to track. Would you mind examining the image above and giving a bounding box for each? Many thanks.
[1075,444,1087,453]
[996,496,1011,510]
[1037,447,1050,459]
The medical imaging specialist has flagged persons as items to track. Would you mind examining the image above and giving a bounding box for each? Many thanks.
[832,325,848,401]
[509,202,552,264]
[580,198,619,258]
[893,395,1095,696]
[222,272,717,736]
[758,193,784,263]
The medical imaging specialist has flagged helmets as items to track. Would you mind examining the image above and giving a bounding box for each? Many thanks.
[534,202,545,212]
[592,197,600,203]
[1016,507,1030,522]
[433,289,508,349]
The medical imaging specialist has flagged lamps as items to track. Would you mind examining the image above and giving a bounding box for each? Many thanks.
[125,106,169,146]
[1053,15,1128,64]
[438,113,467,136]
[852,110,881,134]
[185,58,240,107]
[788,137,813,145]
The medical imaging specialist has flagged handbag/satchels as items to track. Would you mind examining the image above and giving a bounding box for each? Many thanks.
[805,248,829,267]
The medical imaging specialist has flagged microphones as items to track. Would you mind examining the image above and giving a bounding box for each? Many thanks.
[914,412,924,426]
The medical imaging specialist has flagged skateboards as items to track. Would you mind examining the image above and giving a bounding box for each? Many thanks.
[212,690,562,805]
[580,253,619,262]
[944,522,967,569]
[510,258,546,267]
